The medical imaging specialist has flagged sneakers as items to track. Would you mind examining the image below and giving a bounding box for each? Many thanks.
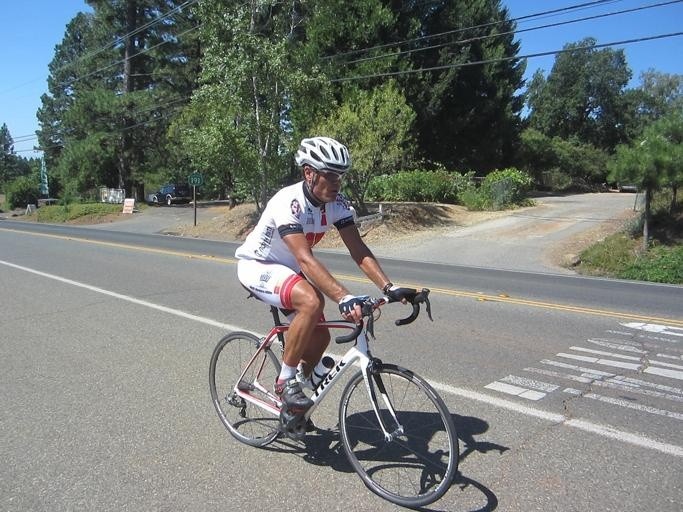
[272,375,314,408]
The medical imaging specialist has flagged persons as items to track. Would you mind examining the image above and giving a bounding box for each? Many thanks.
[235,136,417,432]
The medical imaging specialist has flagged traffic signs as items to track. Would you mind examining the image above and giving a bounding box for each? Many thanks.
[189,173,203,186]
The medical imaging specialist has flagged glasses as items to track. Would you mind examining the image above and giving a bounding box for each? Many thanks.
[318,171,347,182]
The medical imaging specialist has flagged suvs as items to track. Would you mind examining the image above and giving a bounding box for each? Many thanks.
[153,184,193,206]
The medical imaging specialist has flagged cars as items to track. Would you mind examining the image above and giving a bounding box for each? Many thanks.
[617,182,638,193]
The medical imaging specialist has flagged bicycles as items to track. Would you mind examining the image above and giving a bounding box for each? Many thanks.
[209,287,460,508]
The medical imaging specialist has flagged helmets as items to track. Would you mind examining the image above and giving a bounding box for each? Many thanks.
[293,136,350,171]
